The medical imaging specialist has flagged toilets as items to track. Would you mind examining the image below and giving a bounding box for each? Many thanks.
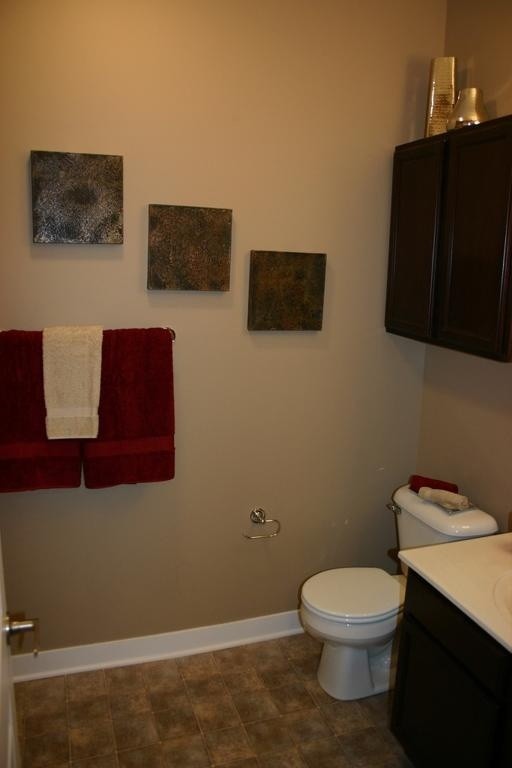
[301,485,498,702]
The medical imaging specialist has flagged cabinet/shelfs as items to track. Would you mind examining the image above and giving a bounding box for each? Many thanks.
[385,115,511,365]
[388,568,511,768]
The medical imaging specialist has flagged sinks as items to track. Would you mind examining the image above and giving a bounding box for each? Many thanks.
[493,568,511,625]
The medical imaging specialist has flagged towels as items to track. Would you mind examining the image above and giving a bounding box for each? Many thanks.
[42,325,103,441]
[82,327,176,487]
[410,475,458,497]
[1,329,82,493]
[418,487,470,512]
